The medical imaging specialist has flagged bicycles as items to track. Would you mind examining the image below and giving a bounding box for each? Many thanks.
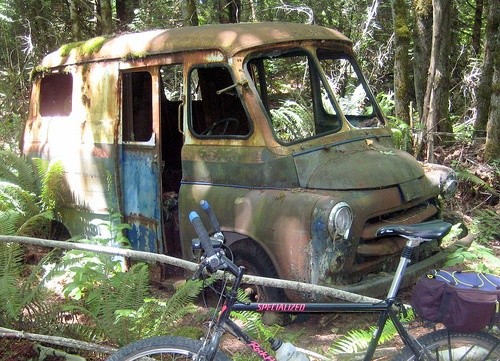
[105,201,500,361]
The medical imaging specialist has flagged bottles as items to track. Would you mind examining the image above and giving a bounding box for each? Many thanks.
[268,336,309,361]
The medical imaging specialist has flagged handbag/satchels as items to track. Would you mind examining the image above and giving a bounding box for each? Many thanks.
[413,268,500,333]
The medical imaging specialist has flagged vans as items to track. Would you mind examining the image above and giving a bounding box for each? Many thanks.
[15,23,477,327]
